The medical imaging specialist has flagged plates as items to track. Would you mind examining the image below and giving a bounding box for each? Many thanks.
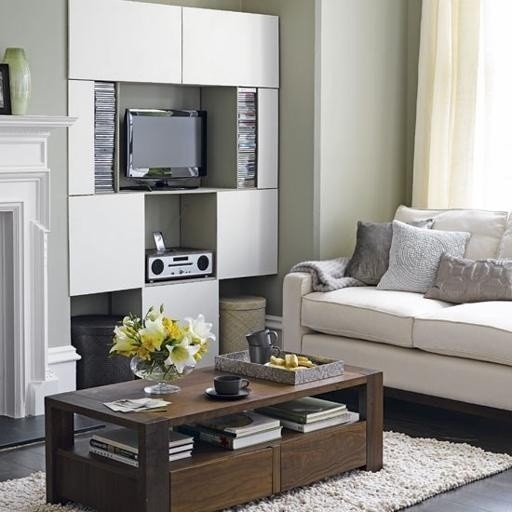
[215,349,344,385]
[204,387,250,400]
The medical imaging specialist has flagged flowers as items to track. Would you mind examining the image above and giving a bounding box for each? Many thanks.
[110,303,216,380]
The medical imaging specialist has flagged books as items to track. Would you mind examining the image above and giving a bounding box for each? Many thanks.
[89,425,195,467]
[173,410,284,449]
[260,397,360,435]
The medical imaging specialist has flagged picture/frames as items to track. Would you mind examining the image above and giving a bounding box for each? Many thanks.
[0,63,12,116]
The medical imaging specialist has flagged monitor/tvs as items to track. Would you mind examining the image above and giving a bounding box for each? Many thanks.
[125,107,207,191]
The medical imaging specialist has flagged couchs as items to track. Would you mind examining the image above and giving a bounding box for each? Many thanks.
[280,202,512,421]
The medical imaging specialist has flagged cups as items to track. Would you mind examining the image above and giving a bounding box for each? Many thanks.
[214,375,250,395]
[245,329,278,346]
[248,345,280,364]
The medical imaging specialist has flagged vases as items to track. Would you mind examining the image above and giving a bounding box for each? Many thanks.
[129,354,194,395]
[4,47,32,115]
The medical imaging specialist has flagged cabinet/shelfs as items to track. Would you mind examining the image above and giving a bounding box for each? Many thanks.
[68,1,279,373]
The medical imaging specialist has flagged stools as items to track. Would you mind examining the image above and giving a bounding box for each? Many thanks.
[221,295,266,353]
[72,313,134,387]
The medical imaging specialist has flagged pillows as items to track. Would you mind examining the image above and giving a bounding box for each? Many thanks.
[344,219,434,285]
[376,219,471,293]
[423,252,512,303]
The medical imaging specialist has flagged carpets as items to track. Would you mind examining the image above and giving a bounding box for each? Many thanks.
[0,426,512,512]
